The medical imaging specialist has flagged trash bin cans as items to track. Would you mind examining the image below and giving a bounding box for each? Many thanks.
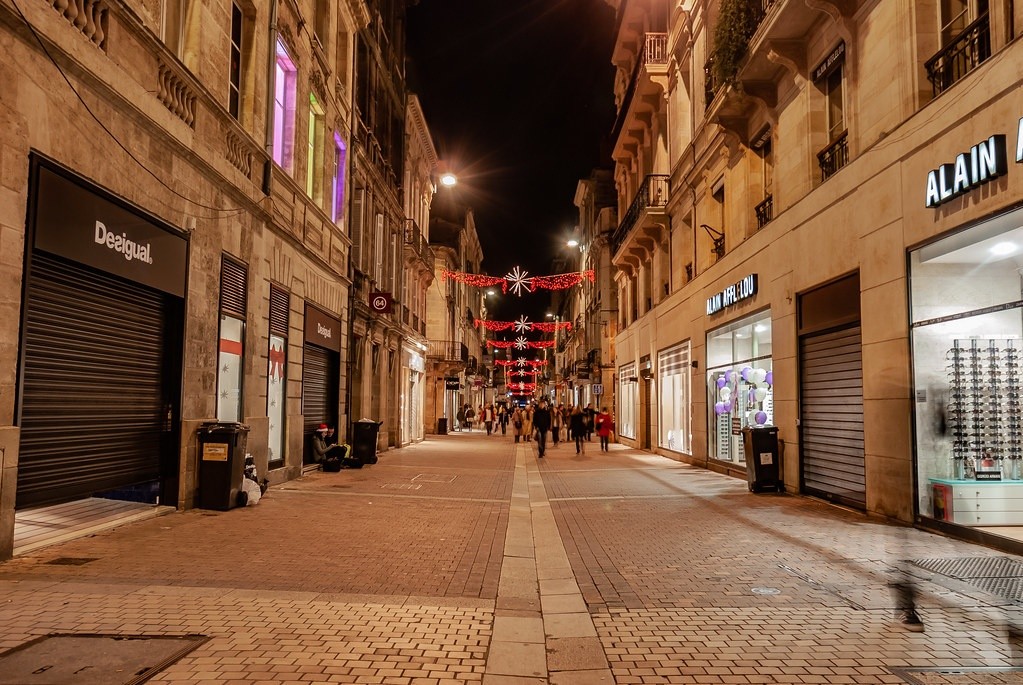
[438,418,448,435]
[196,420,251,510]
[739,424,784,493]
[352,420,384,464]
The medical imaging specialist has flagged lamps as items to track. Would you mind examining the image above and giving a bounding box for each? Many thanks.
[565,226,585,253]
[431,167,457,194]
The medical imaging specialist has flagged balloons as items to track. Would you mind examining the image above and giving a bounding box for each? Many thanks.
[715,366,772,425]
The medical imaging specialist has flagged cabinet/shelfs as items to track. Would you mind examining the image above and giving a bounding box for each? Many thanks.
[928,478,1023,526]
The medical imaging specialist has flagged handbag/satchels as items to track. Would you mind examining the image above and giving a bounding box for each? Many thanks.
[547,431,552,442]
[320,456,342,472]
[515,420,522,429]
[609,430,614,443]
[534,430,541,442]
[595,423,602,431]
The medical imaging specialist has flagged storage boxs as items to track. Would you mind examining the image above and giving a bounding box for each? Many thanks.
[323,460,341,472]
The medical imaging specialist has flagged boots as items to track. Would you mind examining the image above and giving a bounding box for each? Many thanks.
[527,435,532,442]
[515,435,520,443]
[523,435,526,441]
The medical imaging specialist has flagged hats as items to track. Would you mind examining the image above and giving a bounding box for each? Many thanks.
[316,423,328,432]
[526,405,530,410]
[485,404,491,407]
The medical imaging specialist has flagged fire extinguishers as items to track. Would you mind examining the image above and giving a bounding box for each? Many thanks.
[160,402,172,432]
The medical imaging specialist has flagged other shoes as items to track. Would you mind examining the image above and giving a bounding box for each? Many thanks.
[538,453,543,458]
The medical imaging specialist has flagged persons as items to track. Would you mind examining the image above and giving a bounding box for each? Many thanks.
[457,400,612,458]
[313,423,348,472]
